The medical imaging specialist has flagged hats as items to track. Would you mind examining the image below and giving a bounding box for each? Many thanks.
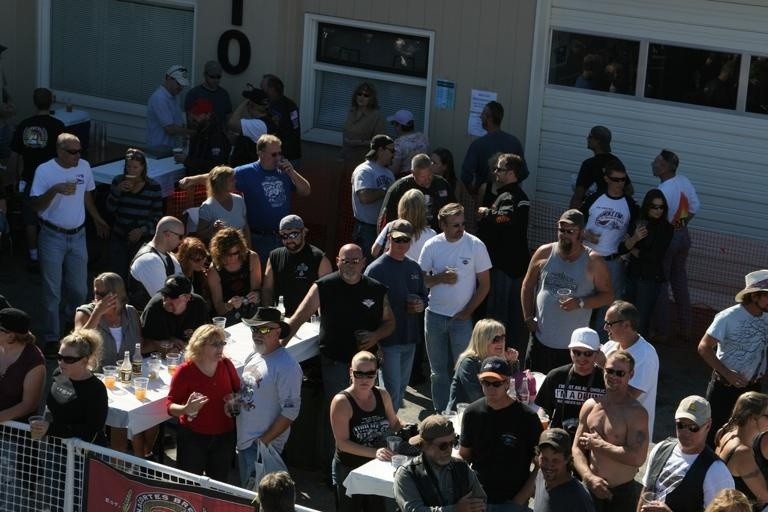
[33,87,53,105]
[408,414,454,447]
[537,428,572,455]
[386,109,415,127]
[558,209,586,230]
[566,327,601,351]
[241,88,268,103]
[674,396,711,426]
[591,126,613,152]
[735,270,767,303]
[278,215,306,233]
[388,219,414,242]
[477,357,511,380]
[157,274,193,296]
[190,99,213,113]
[0,308,30,334]
[241,306,292,341]
[167,65,189,86]
[204,60,222,76]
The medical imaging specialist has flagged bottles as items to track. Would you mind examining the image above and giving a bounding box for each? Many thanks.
[507,379,517,402]
[121,351,132,387]
[132,343,142,375]
[519,377,529,404]
[276,295,285,320]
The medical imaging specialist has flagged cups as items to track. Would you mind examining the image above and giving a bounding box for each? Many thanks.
[557,288,572,302]
[116,360,125,379]
[591,228,602,244]
[408,294,417,314]
[167,352,180,375]
[641,491,659,508]
[133,377,149,401]
[224,394,241,417]
[66,177,77,195]
[145,357,160,378]
[126,175,136,190]
[387,434,402,453]
[213,317,226,331]
[28,415,45,442]
[392,455,407,470]
[355,330,368,346]
[457,403,470,423]
[447,264,462,285]
[442,410,456,429]
[103,366,117,390]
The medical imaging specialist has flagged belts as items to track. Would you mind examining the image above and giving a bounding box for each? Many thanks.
[601,253,621,259]
[39,218,88,235]
[355,218,380,229]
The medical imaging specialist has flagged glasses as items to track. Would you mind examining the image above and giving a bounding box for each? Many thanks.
[337,257,364,265]
[675,420,710,432]
[392,238,411,245]
[249,325,280,335]
[205,341,226,347]
[57,144,85,156]
[480,112,490,119]
[351,369,379,380]
[126,149,144,160]
[356,91,369,97]
[607,176,628,184]
[605,317,632,328]
[160,291,189,302]
[168,67,188,76]
[56,351,84,365]
[225,251,239,256]
[443,222,466,227]
[648,202,667,209]
[384,146,398,154]
[93,289,109,297]
[430,438,460,450]
[385,146,395,152]
[760,411,768,418]
[604,367,631,378]
[488,333,508,343]
[190,257,208,262]
[162,230,187,242]
[558,226,581,233]
[478,379,510,388]
[392,121,395,124]
[570,349,601,357]
[495,165,511,173]
[279,232,306,240]
[263,151,283,156]
[206,75,221,79]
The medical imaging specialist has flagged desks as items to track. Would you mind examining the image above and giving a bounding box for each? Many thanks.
[42,106,95,131]
[88,145,186,199]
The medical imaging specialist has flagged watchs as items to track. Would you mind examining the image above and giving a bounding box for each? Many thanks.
[579,298,585,309]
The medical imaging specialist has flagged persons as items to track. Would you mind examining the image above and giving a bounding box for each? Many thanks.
[336,80,384,168]
[329,351,401,512]
[235,134,311,271]
[459,356,544,512]
[141,274,211,359]
[461,100,523,206]
[595,301,659,444]
[570,351,649,512]
[261,214,332,317]
[145,65,189,159]
[418,202,493,413]
[7,88,65,262]
[371,188,437,264]
[391,413,488,512]
[165,324,241,484]
[520,208,613,378]
[530,428,596,512]
[349,134,396,255]
[697,269,768,432]
[0,43,16,170]
[176,97,232,176]
[478,154,530,318]
[572,54,603,90]
[636,395,734,512]
[184,60,232,130]
[280,243,396,399]
[377,154,455,233]
[28,133,110,348]
[363,219,429,414]
[650,149,701,320]
[533,327,606,443]
[29,328,109,512]
[753,431,768,481]
[75,272,143,373]
[702,62,737,106]
[0,307,46,492]
[232,307,303,488]
[705,488,752,512]
[127,216,185,312]
[206,227,261,319]
[195,165,251,248]
[258,470,297,512]
[714,390,768,506]
[579,163,639,314]
[386,110,428,180]
[259,73,301,141]
[228,88,302,169]
[446,319,520,414]
[176,236,210,299]
[106,147,162,276]
[568,125,634,221]
[619,189,674,342]
[430,148,457,192]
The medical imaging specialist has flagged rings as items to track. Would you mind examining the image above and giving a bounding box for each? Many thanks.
[736,381,740,385]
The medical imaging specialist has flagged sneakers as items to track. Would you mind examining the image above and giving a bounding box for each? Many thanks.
[28,247,37,260]
